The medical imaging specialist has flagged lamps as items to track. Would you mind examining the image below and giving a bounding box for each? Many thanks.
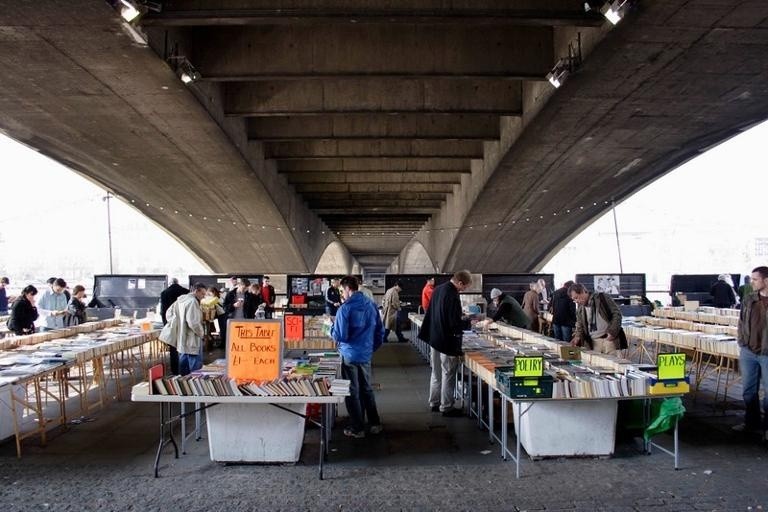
[120,1,142,24]
[176,58,202,86]
[599,0,625,28]
[545,31,582,89]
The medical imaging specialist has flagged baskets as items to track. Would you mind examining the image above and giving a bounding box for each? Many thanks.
[494,364,553,398]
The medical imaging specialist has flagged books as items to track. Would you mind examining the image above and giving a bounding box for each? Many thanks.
[1,316,164,384]
[461,301,658,398]
[621,300,742,358]
[149,315,352,396]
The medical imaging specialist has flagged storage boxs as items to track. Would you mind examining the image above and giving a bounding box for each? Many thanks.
[292,294,305,304]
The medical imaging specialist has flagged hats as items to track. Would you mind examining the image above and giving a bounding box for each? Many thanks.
[490,287,502,300]
[361,280,380,290]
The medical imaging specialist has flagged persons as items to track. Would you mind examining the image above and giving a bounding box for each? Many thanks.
[159,275,375,377]
[63,284,87,327]
[11,284,40,337]
[729,265,768,441]
[380,278,409,344]
[325,274,386,438]
[46,277,71,303]
[0,276,16,316]
[711,274,753,310]
[420,274,437,315]
[37,277,73,332]
[416,269,479,417]
[488,279,624,358]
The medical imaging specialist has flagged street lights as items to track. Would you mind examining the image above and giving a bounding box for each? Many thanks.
[102,195,112,275]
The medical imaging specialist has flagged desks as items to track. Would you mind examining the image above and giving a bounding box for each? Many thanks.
[131,348,344,480]
[0,328,170,459]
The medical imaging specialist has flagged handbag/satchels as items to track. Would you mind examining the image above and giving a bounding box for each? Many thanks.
[614,327,628,351]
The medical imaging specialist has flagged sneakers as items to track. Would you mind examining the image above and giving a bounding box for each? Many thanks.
[731,420,768,444]
[429,401,464,417]
[383,338,409,343]
[343,421,383,440]
